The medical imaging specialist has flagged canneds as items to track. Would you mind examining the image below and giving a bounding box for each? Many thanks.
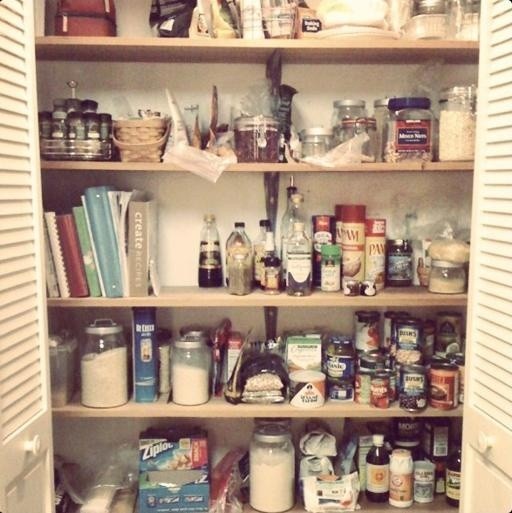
[324,311,465,411]
[385,239,414,287]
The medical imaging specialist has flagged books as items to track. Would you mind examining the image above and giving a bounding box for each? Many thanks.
[45,184,162,301]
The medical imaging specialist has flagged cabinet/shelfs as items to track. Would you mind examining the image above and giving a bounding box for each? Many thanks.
[29,37,482,512]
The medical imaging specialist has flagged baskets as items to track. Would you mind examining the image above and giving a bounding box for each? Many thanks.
[111,116,171,162]
[227,0,297,37]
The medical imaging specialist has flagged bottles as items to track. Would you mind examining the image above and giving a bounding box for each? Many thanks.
[170,329,212,406]
[81,319,129,409]
[427,258,467,294]
[252,416,293,434]
[129,308,158,402]
[153,326,171,395]
[232,113,281,163]
[297,125,333,161]
[198,175,413,297]
[38,97,112,140]
[364,418,461,510]
[248,426,299,512]
[332,83,477,162]
